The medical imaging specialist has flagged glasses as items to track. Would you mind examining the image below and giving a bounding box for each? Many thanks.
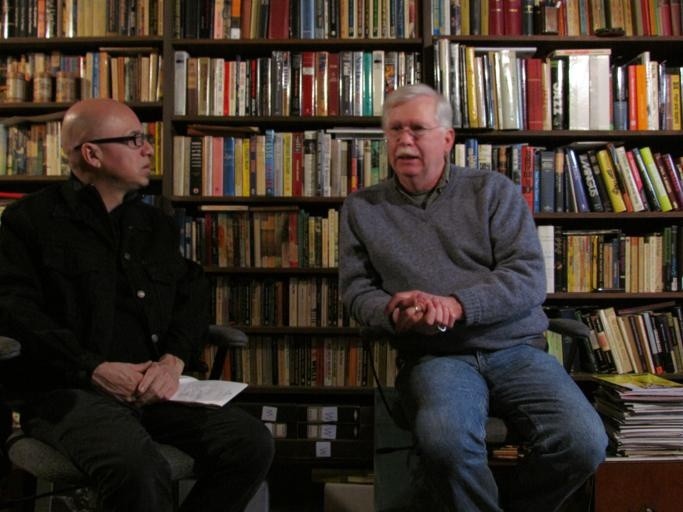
[75,133,155,148]
[386,125,439,137]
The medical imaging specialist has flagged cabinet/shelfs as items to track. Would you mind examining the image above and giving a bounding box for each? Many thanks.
[0,34,166,180]
[426,36,683,512]
[167,38,425,394]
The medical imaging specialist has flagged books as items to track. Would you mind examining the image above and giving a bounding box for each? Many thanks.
[430,0,682,41]
[200,331,396,387]
[0,192,26,216]
[0,112,71,178]
[536,223,682,293]
[173,130,387,197]
[0,0,164,40]
[539,304,682,376]
[175,0,424,43]
[173,51,421,116]
[432,39,683,134]
[583,370,683,461]
[213,277,363,327]
[491,444,531,459]
[0,47,161,103]
[139,121,164,176]
[451,138,683,212]
[174,205,341,270]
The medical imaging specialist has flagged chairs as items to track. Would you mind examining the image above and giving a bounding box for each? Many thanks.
[0,323,252,510]
[361,316,592,511]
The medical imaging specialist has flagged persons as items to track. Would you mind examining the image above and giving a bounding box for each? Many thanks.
[337,80,609,511]
[0,97,278,511]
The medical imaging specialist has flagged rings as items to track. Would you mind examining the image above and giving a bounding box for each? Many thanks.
[434,324,446,333]
[415,306,418,312]
